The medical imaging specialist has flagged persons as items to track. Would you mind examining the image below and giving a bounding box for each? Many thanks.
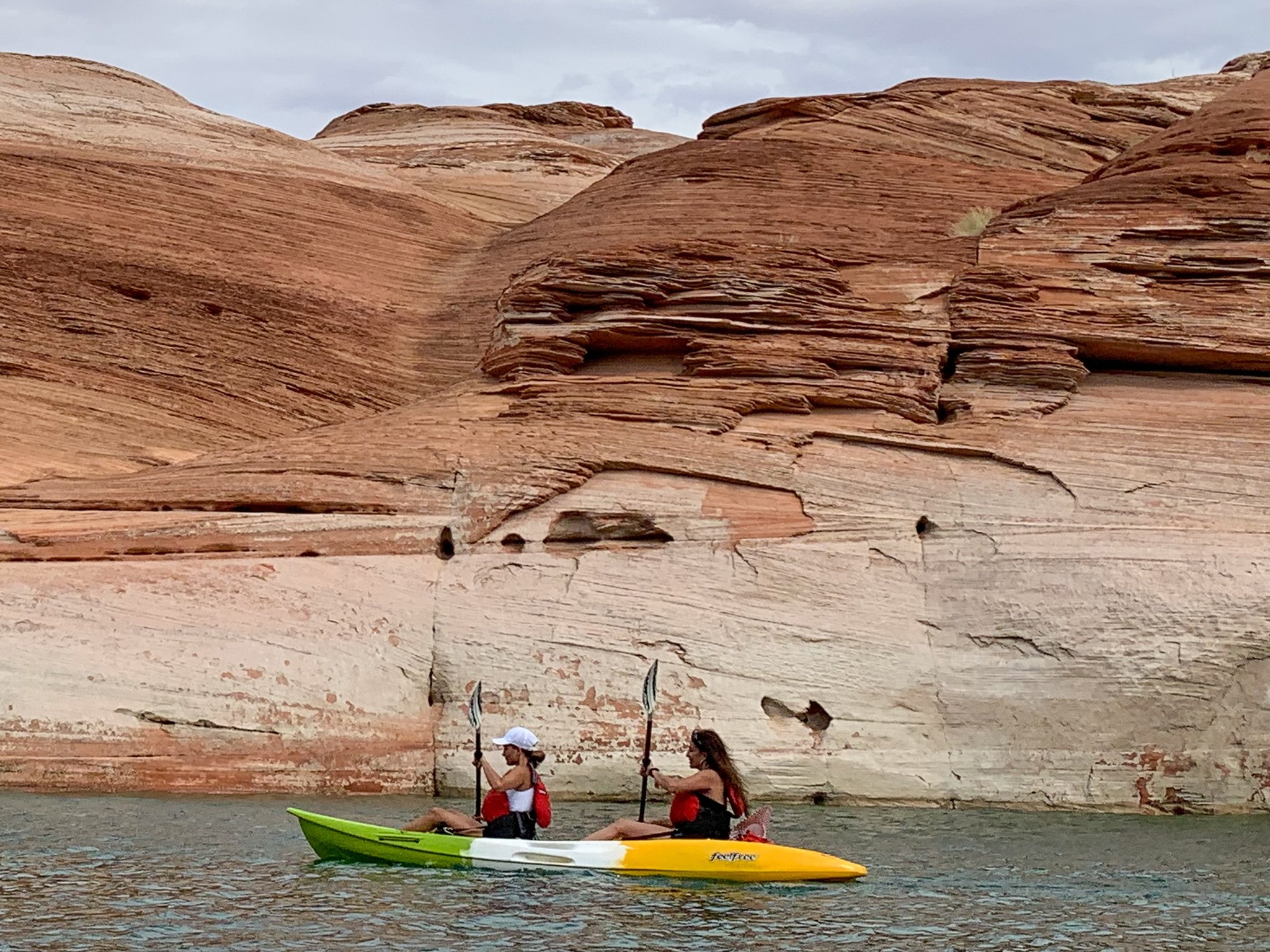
[401,727,551,840]
[583,728,750,840]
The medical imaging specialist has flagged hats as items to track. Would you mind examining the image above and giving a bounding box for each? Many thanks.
[492,727,539,751]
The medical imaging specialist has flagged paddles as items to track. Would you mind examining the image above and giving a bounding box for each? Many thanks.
[638,659,658,822]
[468,680,482,824]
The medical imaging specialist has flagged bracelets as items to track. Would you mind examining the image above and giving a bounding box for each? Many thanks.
[648,768,659,777]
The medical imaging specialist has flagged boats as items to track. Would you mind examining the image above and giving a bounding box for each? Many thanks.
[284,806,869,882]
